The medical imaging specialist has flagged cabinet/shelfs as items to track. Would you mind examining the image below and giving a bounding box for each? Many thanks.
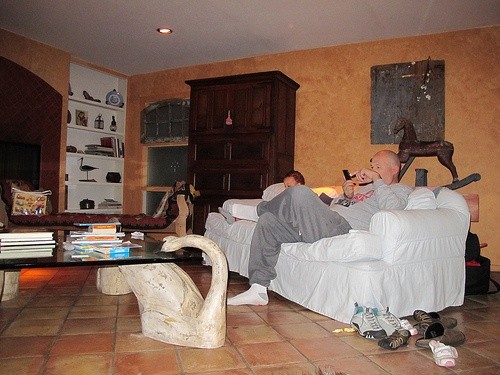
[144,140,188,192]
[185,71,301,255]
[65,61,128,215]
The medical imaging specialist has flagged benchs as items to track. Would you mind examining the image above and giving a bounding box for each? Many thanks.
[0,179,194,242]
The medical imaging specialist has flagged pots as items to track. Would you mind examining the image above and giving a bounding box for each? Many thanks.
[79,198,95,209]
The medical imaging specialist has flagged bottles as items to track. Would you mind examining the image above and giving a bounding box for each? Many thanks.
[94,113,104,130]
[110,115,117,132]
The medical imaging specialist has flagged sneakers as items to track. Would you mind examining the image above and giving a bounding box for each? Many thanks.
[399,318,418,335]
[350,305,387,340]
[429,340,458,368]
[371,306,411,337]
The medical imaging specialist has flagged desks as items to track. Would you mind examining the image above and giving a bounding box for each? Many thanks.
[0,234,229,349]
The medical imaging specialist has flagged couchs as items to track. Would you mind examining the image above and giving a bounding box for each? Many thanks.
[202,187,471,324]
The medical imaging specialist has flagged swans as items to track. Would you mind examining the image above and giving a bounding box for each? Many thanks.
[119,235,230,349]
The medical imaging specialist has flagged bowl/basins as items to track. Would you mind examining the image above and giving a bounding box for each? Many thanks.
[106,172,121,183]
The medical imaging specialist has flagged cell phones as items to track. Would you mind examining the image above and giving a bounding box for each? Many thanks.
[343,170,357,186]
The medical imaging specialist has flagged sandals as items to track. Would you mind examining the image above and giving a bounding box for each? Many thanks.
[413,310,431,331]
[377,329,411,350]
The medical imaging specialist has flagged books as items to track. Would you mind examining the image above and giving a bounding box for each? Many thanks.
[97,199,124,209]
[68,222,142,261]
[0,232,58,258]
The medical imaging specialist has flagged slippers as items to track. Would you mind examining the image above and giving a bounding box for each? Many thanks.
[429,310,457,328]
[416,320,465,349]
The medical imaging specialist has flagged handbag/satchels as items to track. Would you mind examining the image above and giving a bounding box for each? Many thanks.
[465,231,491,295]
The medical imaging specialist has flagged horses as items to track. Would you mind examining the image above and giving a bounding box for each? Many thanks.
[394,116,459,183]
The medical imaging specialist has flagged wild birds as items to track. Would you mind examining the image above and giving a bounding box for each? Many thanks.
[77,157,100,180]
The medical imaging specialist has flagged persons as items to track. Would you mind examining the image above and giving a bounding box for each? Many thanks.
[226,150,415,305]
[284,169,305,190]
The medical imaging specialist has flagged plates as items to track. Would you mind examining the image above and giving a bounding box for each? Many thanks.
[105,90,123,106]
